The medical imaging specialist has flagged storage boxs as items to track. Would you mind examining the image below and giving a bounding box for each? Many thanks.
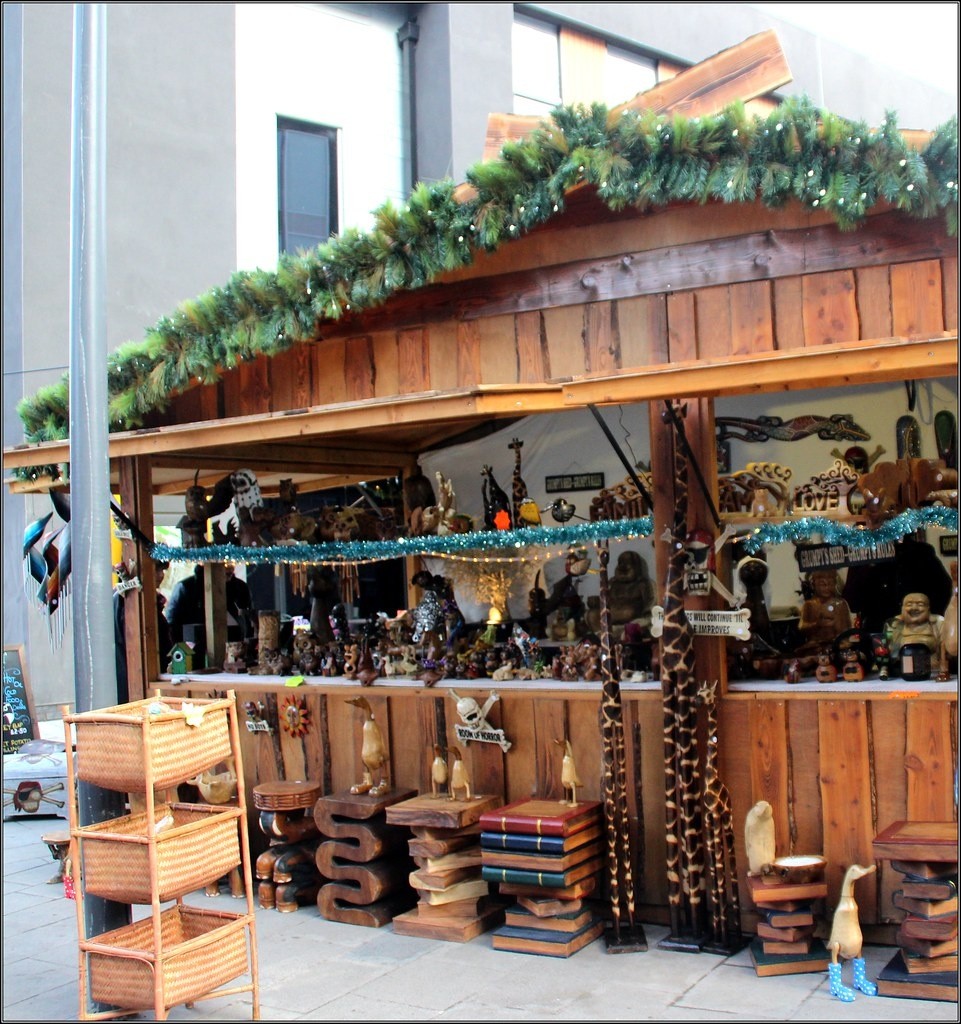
[2,738,78,821]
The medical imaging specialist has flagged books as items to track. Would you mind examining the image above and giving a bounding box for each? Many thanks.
[744,872,836,977]
[870,818,958,1002]
[477,797,604,957]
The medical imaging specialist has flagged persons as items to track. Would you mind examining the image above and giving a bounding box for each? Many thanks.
[108,557,170,708]
[798,567,850,646]
[168,560,253,642]
[887,592,950,665]
[594,550,654,621]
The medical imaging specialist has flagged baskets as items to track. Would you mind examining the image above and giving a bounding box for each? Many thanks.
[81,807,242,905]
[76,701,232,794]
[89,913,249,1010]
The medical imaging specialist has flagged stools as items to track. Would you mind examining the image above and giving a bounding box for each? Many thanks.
[41,830,72,884]
